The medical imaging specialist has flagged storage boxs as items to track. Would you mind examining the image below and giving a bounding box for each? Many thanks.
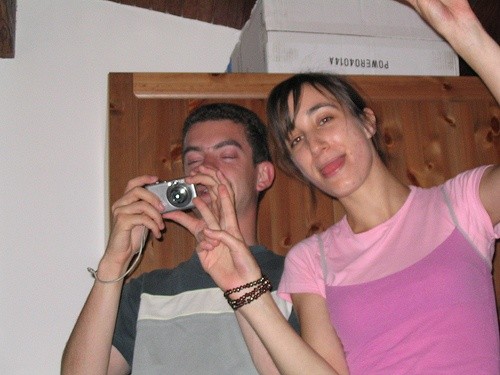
[225,0,460,76]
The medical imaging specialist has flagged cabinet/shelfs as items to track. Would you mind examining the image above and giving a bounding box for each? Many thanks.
[106,72,500,327]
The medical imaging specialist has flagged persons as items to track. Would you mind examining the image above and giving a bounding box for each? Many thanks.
[60,103,300,375]
[193,0,500,375]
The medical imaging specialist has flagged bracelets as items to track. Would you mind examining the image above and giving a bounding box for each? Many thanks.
[223,277,272,310]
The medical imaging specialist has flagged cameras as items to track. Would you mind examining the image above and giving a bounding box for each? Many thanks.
[145,178,198,214]
